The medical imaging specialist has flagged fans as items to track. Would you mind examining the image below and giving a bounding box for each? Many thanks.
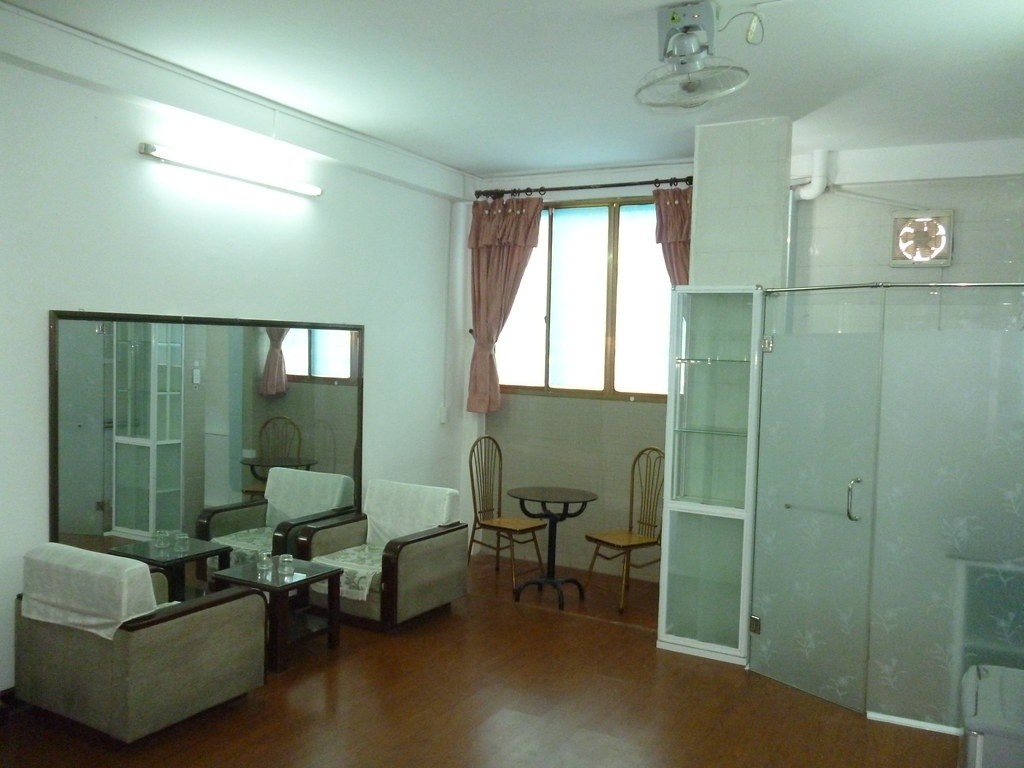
[888,209,954,267]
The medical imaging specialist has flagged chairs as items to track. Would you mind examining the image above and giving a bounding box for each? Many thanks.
[578,446,665,614]
[258,416,301,457]
[467,435,546,594]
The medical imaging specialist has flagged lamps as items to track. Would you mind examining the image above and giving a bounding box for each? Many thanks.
[634,1,765,109]
[138,141,323,197]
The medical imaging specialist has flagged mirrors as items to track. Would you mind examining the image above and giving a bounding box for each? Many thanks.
[48,308,365,583]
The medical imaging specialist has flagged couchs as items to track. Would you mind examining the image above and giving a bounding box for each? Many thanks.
[193,464,354,579]
[294,478,470,634]
[12,543,269,742]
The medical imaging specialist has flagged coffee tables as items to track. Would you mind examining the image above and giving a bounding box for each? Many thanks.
[109,534,234,597]
[241,456,318,485]
[507,486,600,612]
[210,554,345,674]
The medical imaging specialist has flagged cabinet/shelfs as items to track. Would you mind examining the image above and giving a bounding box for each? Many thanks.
[653,286,764,671]
[108,320,187,543]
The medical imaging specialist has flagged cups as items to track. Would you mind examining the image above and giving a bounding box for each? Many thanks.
[278,555,295,576]
[174,533,189,552]
[257,550,272,570]
[155,530,170,549]
